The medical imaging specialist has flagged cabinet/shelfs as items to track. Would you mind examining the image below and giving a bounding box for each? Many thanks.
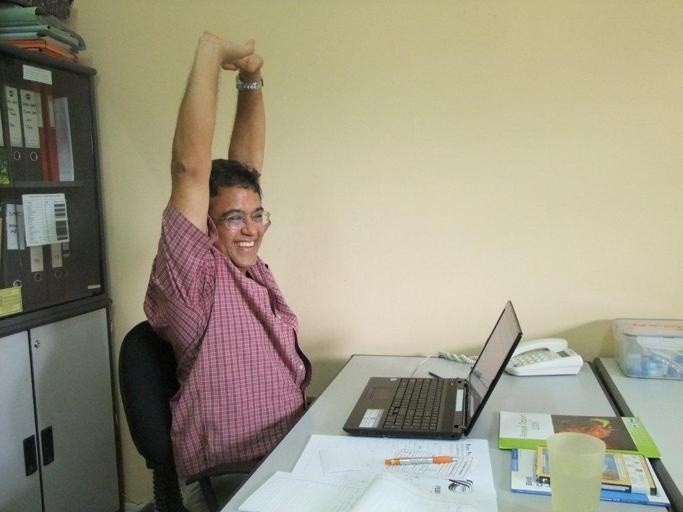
[0,298,124,512]
[0,45,109,319]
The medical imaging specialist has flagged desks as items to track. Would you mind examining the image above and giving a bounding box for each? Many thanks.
[220,354,670,512]
[593,357,683,512]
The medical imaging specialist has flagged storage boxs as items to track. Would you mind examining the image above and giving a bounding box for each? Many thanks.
[614,319,683,380]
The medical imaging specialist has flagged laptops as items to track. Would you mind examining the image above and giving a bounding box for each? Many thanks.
[342,300,523,439]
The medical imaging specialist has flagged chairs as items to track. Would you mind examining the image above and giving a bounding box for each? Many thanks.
[118,321,315,510]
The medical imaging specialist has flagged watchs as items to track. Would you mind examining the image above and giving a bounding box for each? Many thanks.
[236,73,264,92]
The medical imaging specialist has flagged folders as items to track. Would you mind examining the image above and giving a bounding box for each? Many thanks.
[0,76,44,182]
[0,202,68,315]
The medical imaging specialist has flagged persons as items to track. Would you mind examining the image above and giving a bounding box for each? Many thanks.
[144,28,314,512]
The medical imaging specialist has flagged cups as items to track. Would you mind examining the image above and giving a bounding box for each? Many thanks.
[547,432,607,512]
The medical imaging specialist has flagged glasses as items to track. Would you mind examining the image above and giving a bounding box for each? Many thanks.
[208,209,271,232]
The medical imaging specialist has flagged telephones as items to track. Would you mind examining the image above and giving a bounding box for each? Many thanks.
[504,337,584,377]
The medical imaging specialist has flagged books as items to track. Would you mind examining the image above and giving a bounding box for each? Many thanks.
[499,411,672,508]
[0,0,88,312]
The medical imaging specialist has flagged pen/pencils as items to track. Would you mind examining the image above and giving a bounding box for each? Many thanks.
[384,456,459,466]
